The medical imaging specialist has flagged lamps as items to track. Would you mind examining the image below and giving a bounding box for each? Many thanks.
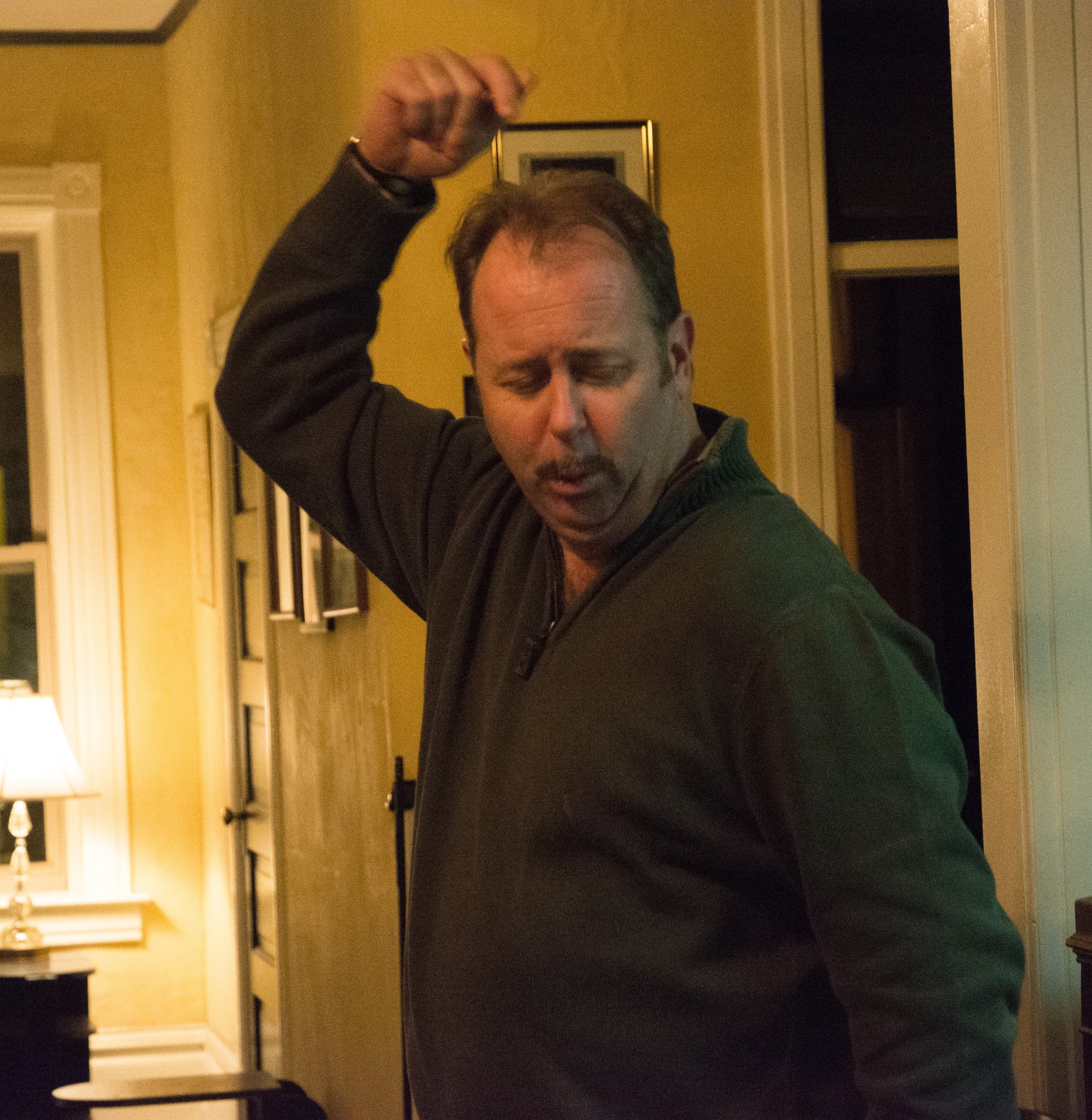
[0,691,98,947]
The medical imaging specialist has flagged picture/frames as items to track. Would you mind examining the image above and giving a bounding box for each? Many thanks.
[185,403,217,608]
[263,476,301,620]
[319,526,369,619]
[492,114,658,213]
[298,507,335,634]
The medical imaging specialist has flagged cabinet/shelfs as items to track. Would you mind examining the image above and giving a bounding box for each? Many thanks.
[2,950,99,1120]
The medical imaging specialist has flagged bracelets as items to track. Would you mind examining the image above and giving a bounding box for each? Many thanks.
[347,139,434,205]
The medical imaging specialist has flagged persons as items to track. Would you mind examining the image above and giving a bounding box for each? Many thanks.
[210,44,1030,1119]
